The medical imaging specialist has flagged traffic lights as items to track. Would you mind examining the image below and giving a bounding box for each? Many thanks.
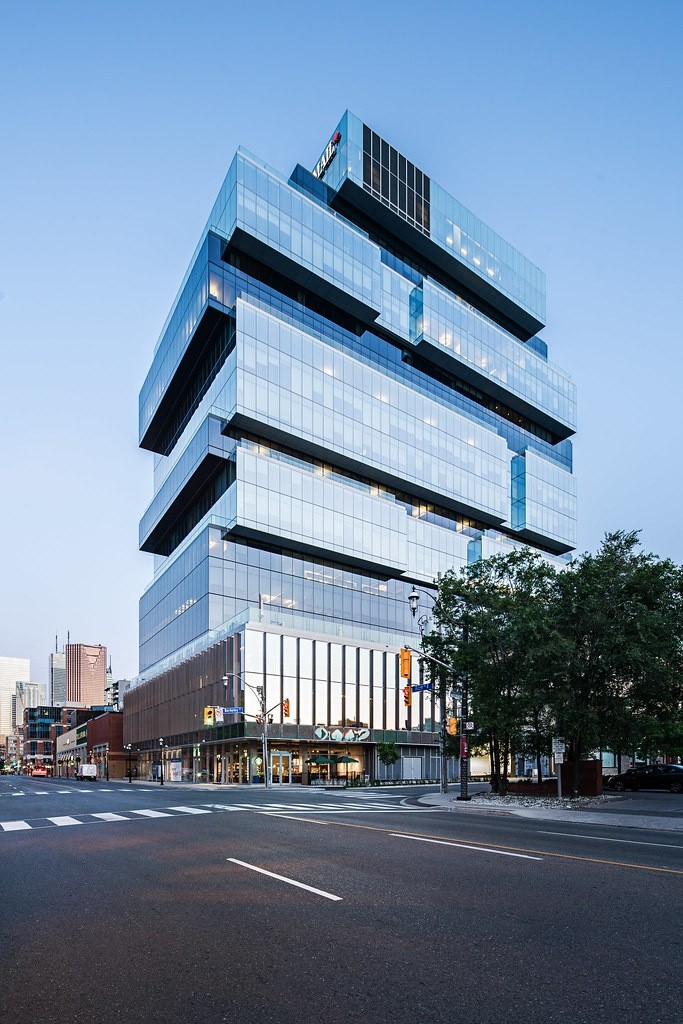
[204,707,214,725]
[402,686,411,707]
[255,714,261,724]
[283,699,290,717]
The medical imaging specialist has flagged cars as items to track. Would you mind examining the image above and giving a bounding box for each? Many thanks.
[607,764,683,794]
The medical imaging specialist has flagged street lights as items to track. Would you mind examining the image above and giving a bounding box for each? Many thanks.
[123,743,133,783]
[224,672,268,789]
[105,747,109,781]
[409,585,472,800]
[159,737,164,786]
[89,750,92,764]
